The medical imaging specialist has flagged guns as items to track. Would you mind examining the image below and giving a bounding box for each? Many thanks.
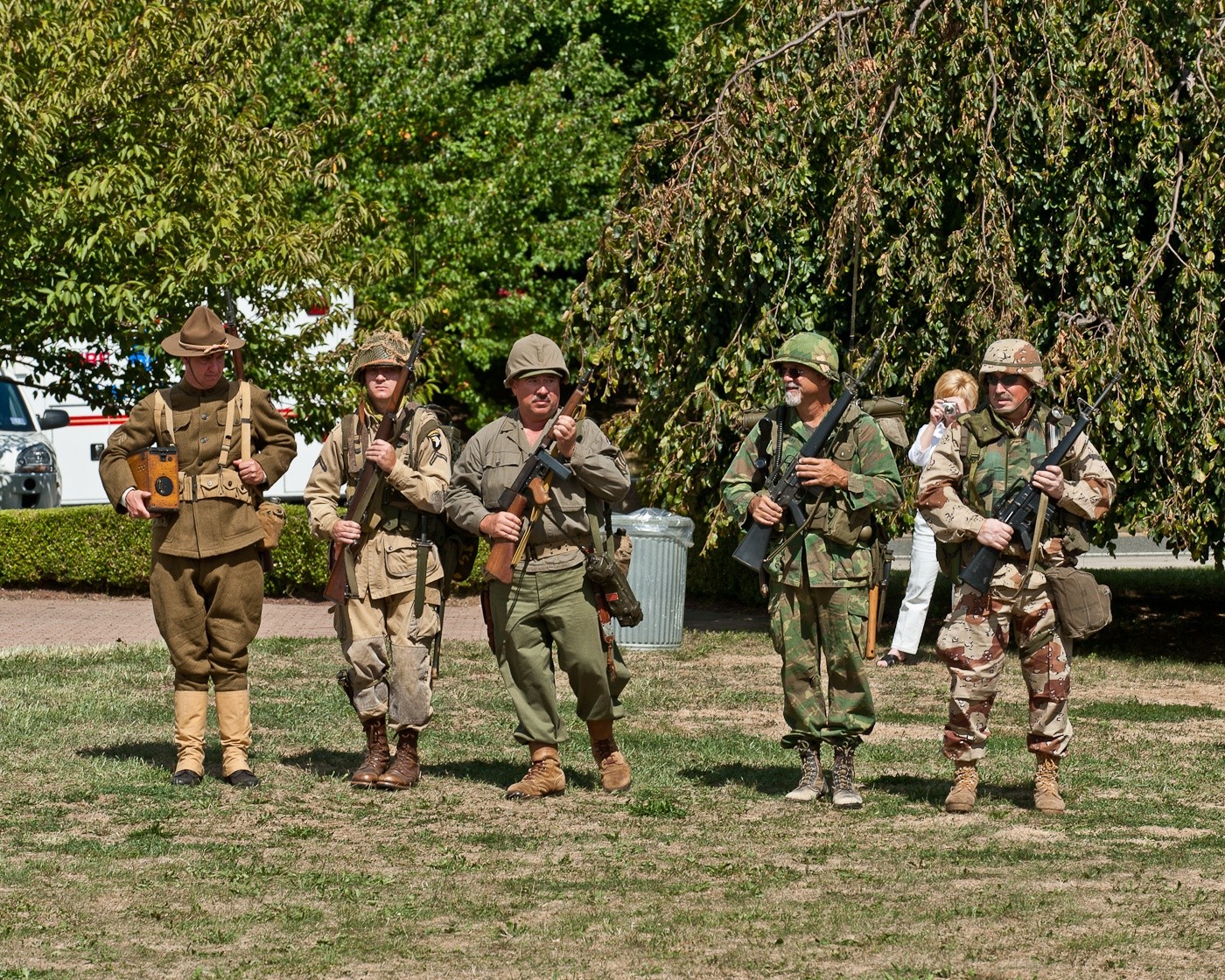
[223,286,245,381]
[323,326,426,607]
[485,368,592,585]
[730,351,882,573]
[959,374,1120,595]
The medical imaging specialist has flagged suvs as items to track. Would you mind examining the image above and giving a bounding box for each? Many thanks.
[0,374,70,508]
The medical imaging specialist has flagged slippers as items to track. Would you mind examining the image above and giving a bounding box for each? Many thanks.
[876,650,905,669]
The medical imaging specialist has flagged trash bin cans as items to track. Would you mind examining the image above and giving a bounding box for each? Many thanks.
[604,515,694,650]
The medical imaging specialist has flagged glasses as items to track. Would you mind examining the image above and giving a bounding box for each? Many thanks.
[987,373,1022,386]
[778,366,818,379]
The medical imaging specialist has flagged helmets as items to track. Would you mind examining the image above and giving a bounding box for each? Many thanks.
[504,335,569,388]
[352,334,416,385]
[979,338,1044,387]
[769,332,840,383]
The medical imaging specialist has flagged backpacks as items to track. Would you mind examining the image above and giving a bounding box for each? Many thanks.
[342,413,478,581]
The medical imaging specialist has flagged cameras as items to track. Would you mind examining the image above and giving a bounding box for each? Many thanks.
[936,402,958,416]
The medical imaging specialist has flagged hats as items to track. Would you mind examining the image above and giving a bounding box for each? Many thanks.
[517,369,563,379]
[160,304,246,357]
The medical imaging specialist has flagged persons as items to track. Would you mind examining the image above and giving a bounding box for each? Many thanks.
[98,307,297,787]
[719,332,904,809]
[876,369,978,667]
[444,333,634,799]
[917,338,1117,813]
[304,331,452,789]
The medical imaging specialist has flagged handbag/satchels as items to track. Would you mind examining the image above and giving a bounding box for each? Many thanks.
[256,500,288,547]
[596,528,633,580]
[1045,566,1113,639]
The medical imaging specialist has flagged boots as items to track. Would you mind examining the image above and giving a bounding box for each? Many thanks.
[944,757,980,813]
[831,745,863,806]
[378,729,421,788]
[1033,748,1065,811]
[171,688,208,787]
[785,743,830,801]
[215,684,258,786]
[585,718,631,792]
[503,737,566,801]
[349,716,392,786]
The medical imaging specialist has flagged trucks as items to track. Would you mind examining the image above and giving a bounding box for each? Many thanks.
[0,278,355,504]
